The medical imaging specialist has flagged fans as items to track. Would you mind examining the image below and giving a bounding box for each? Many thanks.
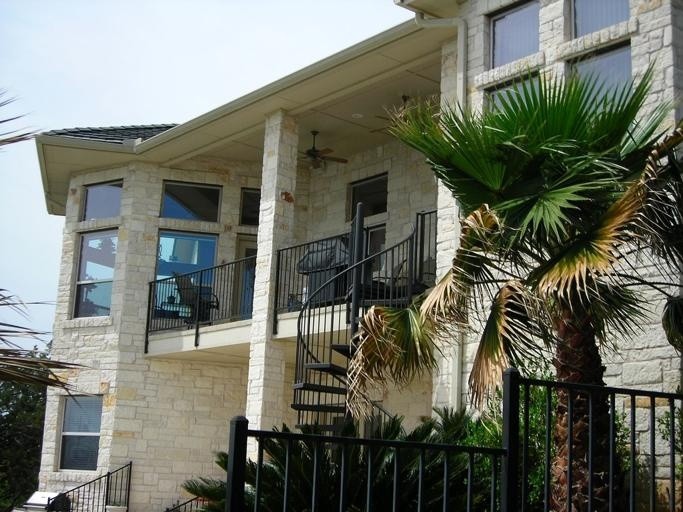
[297,129,348,164]
[370,95,413,135]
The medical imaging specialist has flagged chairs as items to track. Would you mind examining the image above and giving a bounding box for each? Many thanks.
[172,270,219,330]
[344,257,408,303]
[152,293,180,331]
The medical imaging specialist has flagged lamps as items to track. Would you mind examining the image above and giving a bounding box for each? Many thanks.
[311,159,321,169]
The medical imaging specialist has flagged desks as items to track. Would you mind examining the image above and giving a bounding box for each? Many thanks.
[159,301,190,330]
